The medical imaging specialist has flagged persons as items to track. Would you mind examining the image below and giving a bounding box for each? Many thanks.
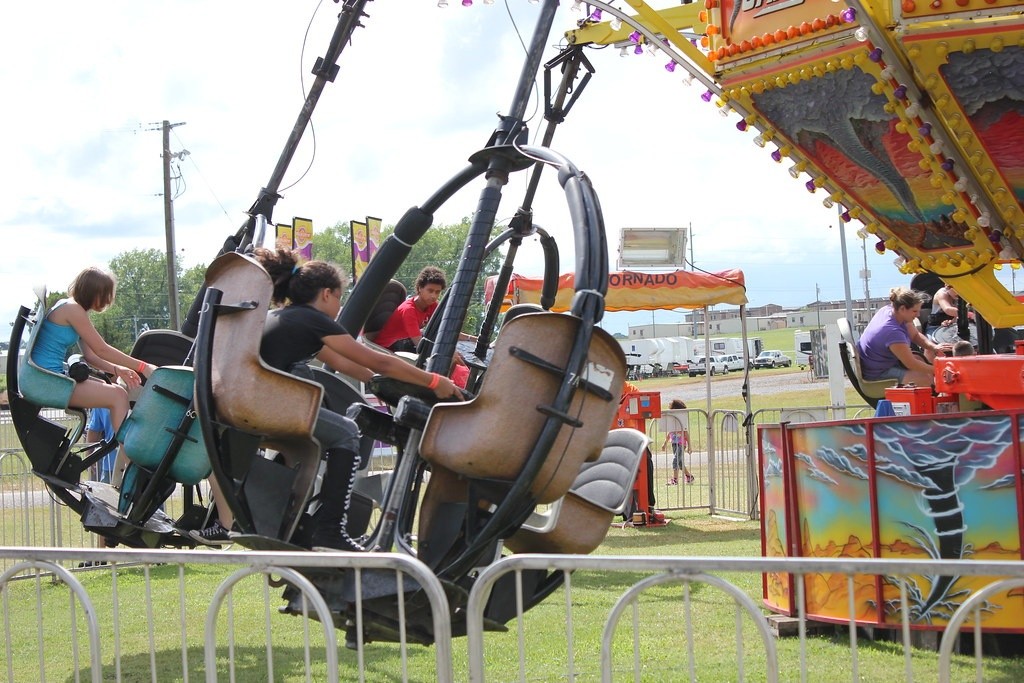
[79,370,168,568]
[189,248,489,559]
[662,400,694,485]
[612,381,657,525]
[30,268,161,473]
[856,283,977,404]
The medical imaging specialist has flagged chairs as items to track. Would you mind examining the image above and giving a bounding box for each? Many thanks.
[836,272,946,414]
[5,250,652,557]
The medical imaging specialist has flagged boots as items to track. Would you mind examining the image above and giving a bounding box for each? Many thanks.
[311,447,372,551]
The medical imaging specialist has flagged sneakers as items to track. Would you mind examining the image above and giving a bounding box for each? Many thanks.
[666,478,678,486]
[684,473,695,485]
[188,521,234,545]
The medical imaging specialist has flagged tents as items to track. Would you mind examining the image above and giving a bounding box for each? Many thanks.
[485,267,759,520]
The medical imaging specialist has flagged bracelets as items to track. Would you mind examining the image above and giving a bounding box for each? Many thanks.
[138,362,146,373]
[467,335,472,341]
[114,364,118,376]
[428,372,439,390]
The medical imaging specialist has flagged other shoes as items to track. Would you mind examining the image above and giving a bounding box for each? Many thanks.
[78,560,108,568]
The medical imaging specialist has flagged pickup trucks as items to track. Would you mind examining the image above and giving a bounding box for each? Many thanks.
[720,354,754,372]
[755,349,792,369]
[688,356,728,377]
[672,361,689,373]
